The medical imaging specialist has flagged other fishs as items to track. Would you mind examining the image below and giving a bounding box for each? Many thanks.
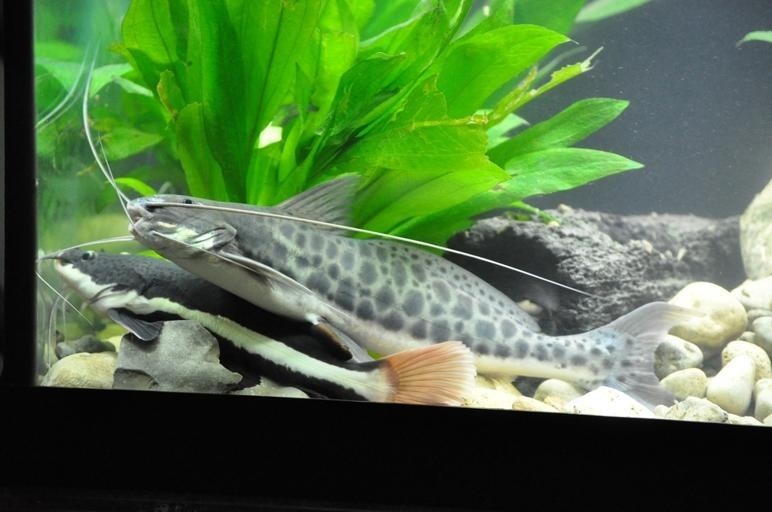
[81,41,692,409]
[34,234,477,408]
